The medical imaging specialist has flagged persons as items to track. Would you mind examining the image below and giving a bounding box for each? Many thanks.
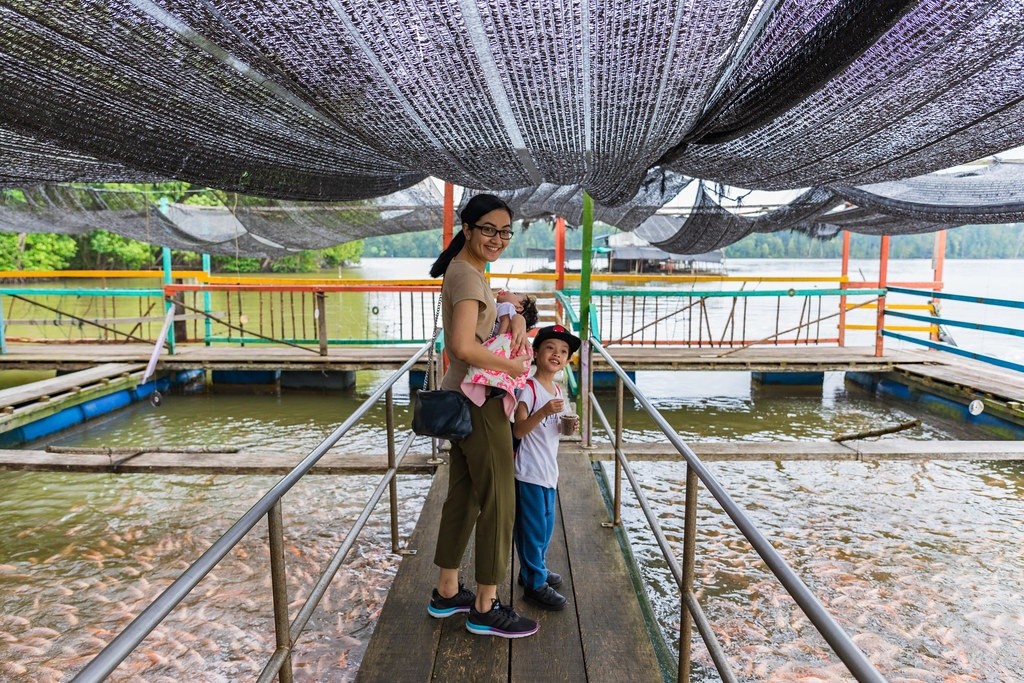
[428,194,539,638]
[512,324,579,608]
[489,287,537,397]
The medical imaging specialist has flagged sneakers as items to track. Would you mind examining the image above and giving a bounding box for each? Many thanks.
[464,599,542,639]
[424,580,477,618]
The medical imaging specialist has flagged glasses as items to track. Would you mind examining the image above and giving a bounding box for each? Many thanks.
[468,222,514,241]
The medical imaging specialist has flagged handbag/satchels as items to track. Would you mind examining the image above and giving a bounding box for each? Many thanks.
[410,388,473,441]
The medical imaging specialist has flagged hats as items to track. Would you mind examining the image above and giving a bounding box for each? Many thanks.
[534,324,582,355]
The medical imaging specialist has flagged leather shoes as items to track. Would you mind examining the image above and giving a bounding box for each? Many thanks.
[523,581,567,613]
[514,565,563,590]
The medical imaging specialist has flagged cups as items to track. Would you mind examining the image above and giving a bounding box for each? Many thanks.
[560,414,579,436]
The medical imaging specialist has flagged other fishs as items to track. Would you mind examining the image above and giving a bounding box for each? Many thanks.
[636,474,1024,683]
[0,493,430,683]
